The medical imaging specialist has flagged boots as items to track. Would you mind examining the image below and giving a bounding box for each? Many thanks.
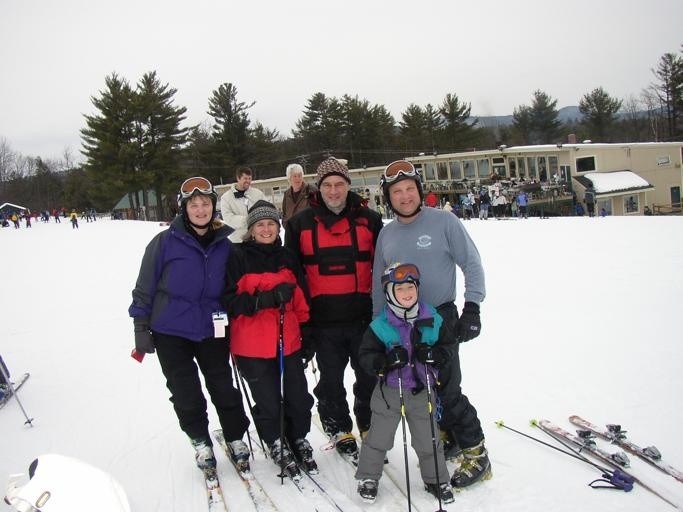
[449,438,492,488]
[438,429,463,455]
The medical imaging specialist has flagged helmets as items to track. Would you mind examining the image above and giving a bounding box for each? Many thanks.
[378,170,423,200]
[177,184,218,210]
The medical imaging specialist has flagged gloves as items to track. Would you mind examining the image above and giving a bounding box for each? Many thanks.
[297,322,316,369]
[418,345,447,366]
[456,300,481,343]
[384,344,410,371]
[134,321,156,354]
[259,281,297,312]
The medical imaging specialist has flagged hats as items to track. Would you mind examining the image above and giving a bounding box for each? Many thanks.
[315,156,352,189]
[246,199,282,228]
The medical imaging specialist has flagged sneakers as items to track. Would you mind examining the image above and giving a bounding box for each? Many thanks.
[331,432,358,457]
[424,481,453,501]
[290,437,315,469]
[192,441,217,473]
[228,440,252,468]
[268,436,296,473]
[358,476,379,500]
[360,430,387,456]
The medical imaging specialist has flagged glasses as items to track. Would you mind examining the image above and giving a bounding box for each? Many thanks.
[180,176,213,198]
[388,263,421,283]
[383,160,417,183]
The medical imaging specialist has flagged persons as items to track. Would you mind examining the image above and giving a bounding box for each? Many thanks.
[220,167,267,243]
[358,161,652,220]
[285,157,383,453]
[0,206,144,229]
[222,200,318,471]
[282,163,319,230]
[127,177,251,468]
[354,262,459,502]
[0,354,11,403]
[372,159,490,487]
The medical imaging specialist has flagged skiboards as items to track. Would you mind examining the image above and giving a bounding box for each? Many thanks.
[247,428,362,512]
[201,416,683,512]
[312,415,438,512]
[202,429,278,512]
[541,416,683,511]
[0,372,30,407]
